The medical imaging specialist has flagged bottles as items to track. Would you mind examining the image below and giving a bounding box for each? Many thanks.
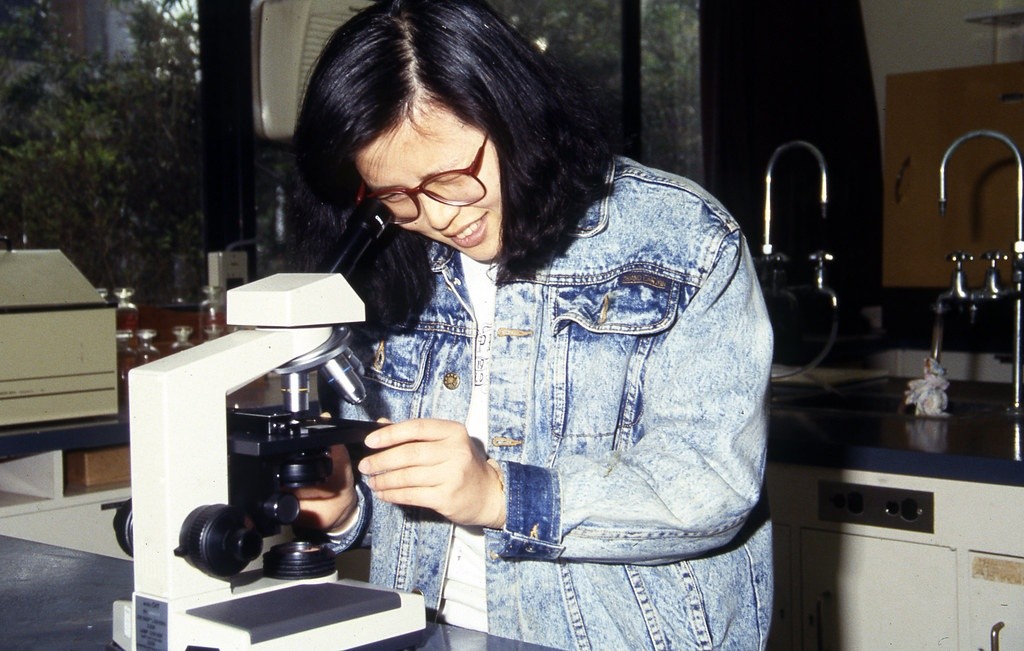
[201,286,228,343]
[167,325,194,356]
[134,328,162,366]
[116,328,137,403]
[110,287,138,334]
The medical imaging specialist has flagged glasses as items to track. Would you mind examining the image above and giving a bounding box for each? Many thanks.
[354,133,489,225]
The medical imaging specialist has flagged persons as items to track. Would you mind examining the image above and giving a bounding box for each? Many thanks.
[275,0,774,651]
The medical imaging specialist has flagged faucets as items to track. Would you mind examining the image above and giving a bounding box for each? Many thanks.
[761,139,829,282]
[970,248,1015,322]
[757,252,799,309]
[938,129,1024,417]
[930,250,983,315]
[789,249,842,311]
